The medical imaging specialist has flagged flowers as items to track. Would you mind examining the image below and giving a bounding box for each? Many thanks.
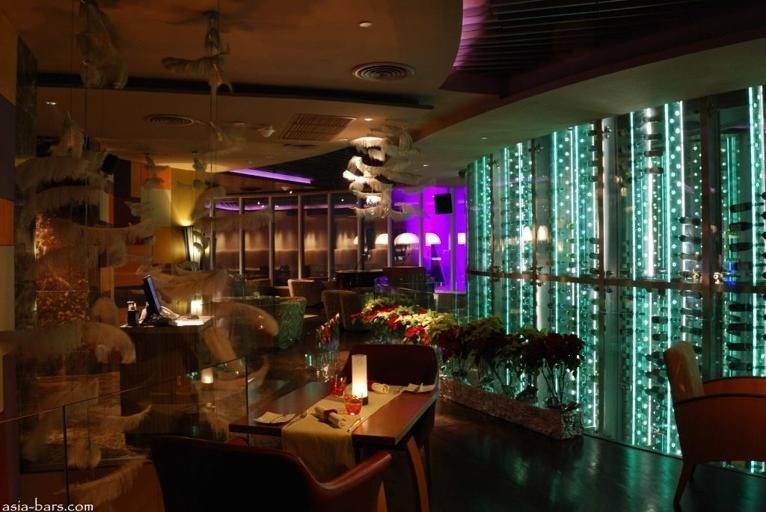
[430,313,585,410]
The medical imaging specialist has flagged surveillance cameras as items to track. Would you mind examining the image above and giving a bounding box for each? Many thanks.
[458,169,468,178]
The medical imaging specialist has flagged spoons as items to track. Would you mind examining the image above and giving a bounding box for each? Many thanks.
[270,409,290,423]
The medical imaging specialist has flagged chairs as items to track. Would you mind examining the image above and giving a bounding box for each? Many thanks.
[120,277,439,512]
[663,341,766,506]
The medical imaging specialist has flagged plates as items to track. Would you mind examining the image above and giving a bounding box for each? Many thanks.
[405,383,436,394]
[253,411,297,425]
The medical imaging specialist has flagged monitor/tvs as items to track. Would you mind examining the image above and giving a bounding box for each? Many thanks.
[142,275,160,323]
[434,192,453,214]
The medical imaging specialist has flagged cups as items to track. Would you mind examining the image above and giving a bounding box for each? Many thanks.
[330,374,346,399]
[343,391,363,416]
